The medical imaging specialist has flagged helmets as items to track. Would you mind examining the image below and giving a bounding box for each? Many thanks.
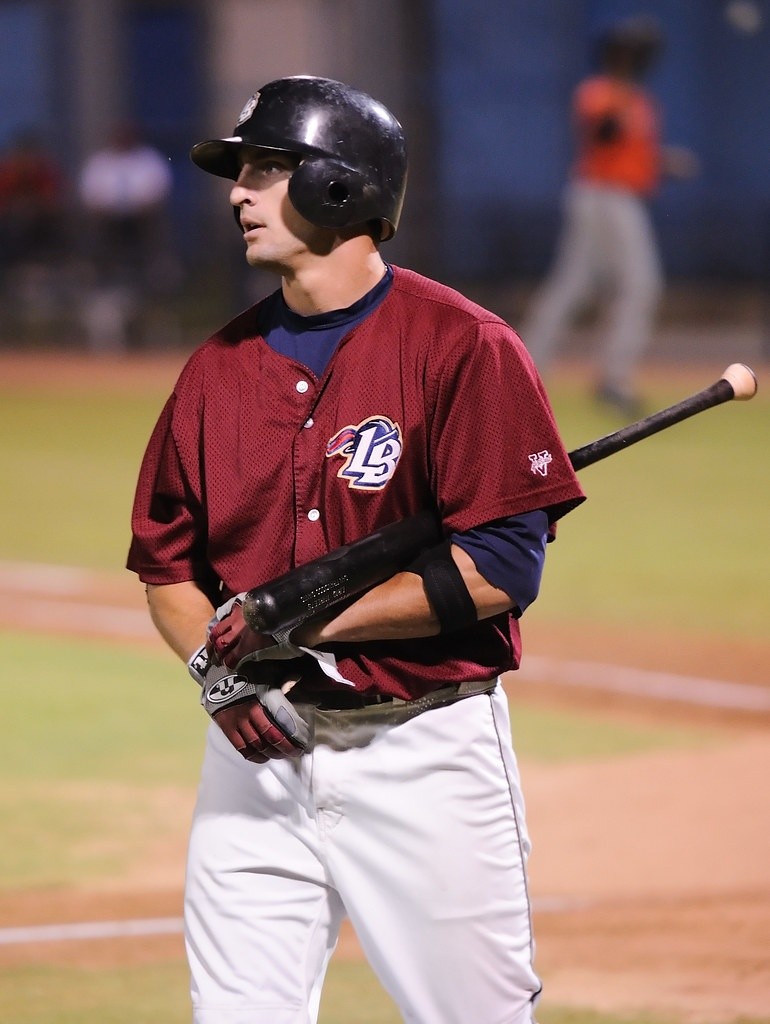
[192,75,407,241]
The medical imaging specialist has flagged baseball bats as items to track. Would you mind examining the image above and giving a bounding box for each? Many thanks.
[247,358,758,638]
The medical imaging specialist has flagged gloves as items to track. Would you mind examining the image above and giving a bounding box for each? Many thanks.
[187,642,313,765]
[207,590,306,672]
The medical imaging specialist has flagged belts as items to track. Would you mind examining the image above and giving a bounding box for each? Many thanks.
[286,690,393,708]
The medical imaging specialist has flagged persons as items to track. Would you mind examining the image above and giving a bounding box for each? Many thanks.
[125,75,588,1024]
[518,15,694,418]
[0,122,172,350]
